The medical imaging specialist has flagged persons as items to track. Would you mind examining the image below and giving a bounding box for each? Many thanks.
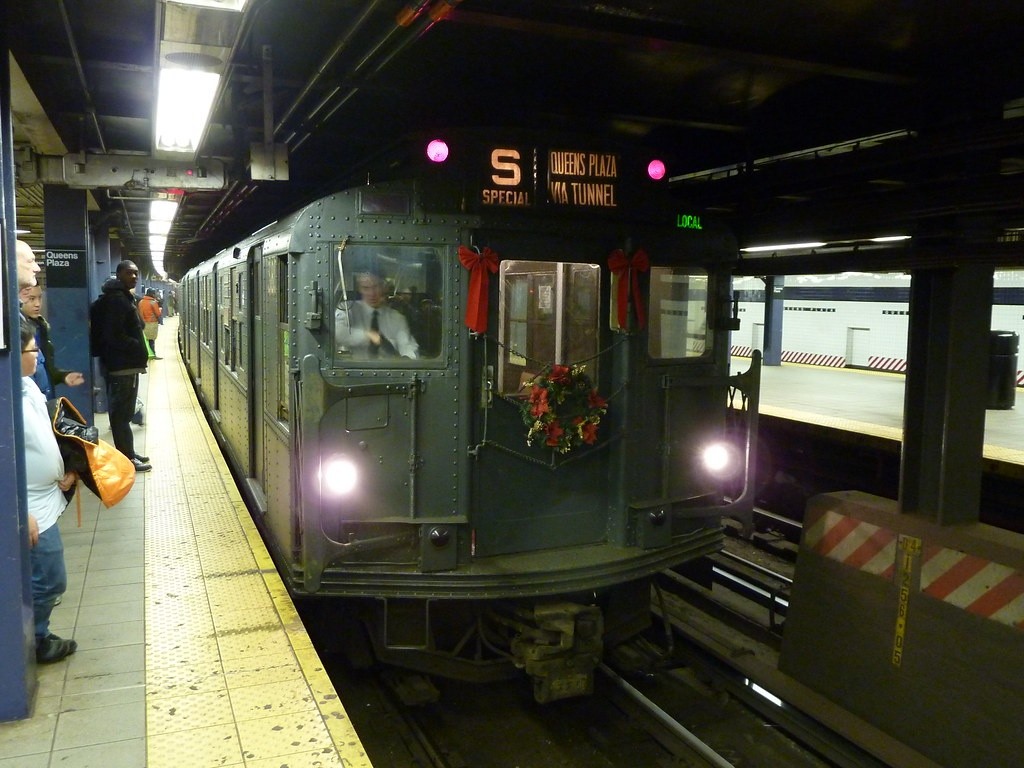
[89,260,152,472]
[334,264,420,360]
[16,239,85,606]
[20,319,79,665]
[166,290,174,317]
[138,287,164,360]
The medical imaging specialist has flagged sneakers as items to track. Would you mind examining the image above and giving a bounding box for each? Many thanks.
[131,454,152,472]
[36,630,77,663]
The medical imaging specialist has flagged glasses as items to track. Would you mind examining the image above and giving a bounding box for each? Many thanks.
[22,348,41,354]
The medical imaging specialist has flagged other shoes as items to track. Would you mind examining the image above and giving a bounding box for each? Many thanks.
[149,355,162,360]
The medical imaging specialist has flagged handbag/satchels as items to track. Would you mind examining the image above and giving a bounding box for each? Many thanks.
[50,390,136,508]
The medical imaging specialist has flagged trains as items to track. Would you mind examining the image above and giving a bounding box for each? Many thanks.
[178,129,762,712]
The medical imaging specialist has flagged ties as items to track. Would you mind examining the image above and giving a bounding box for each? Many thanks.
[368,309,381,358]
